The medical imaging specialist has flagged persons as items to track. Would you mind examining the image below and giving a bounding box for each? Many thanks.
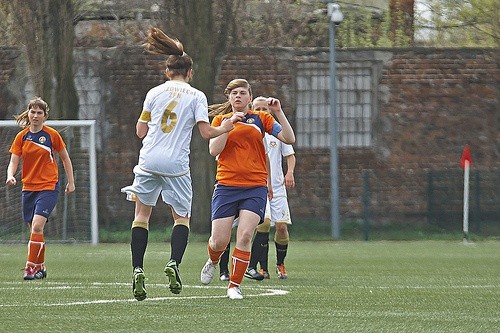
[119,25,235,302]
[200,79,295,299]
[220,214,266,281]
[6,97,76,280]
[251,95,297,279]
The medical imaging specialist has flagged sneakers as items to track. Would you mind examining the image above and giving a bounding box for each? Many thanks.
[219,273,230,281]
[200,258,217,285]
[131,266,147,302]
[275,262,288,280]
[163,258,182,295]
[33,267,47,280]
[244,266,264,281]
[258,268,270,280]
[226,283,244,300]
[23,267,38,280]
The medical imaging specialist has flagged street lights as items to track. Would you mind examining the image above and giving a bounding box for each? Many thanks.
[326,2,345,240]
